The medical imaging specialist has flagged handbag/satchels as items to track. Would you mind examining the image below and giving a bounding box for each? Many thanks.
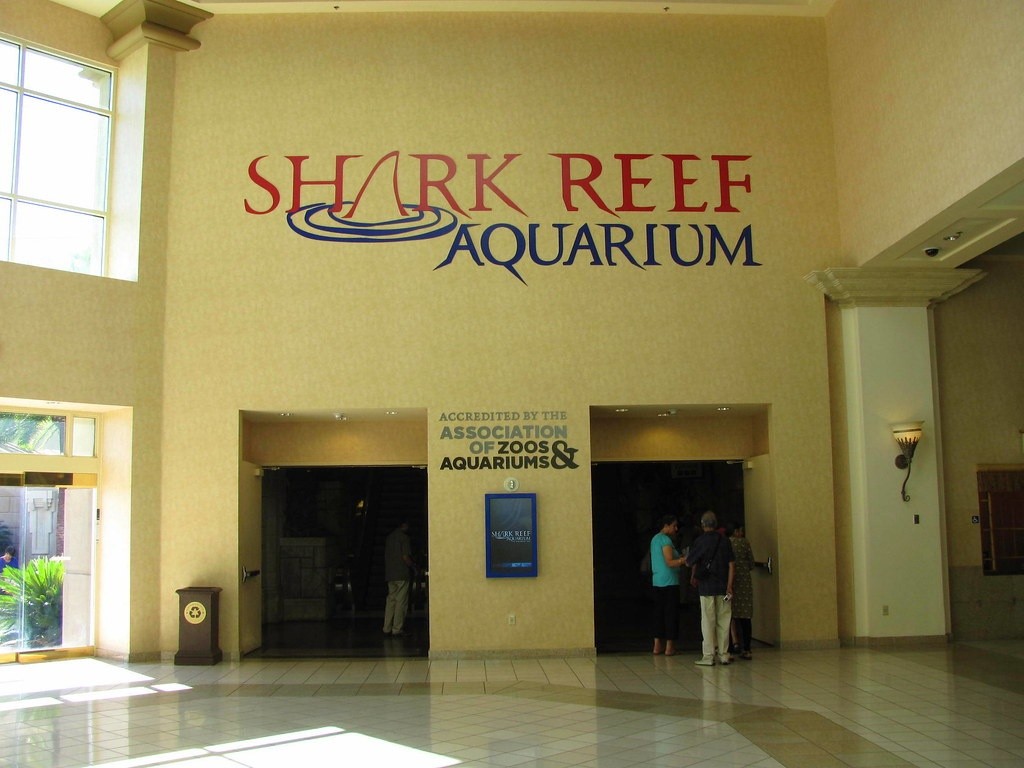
[694,555,729,583]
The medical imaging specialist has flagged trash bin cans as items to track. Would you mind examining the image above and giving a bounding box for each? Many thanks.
[174,586,224,667]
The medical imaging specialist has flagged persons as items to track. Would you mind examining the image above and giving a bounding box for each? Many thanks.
[730,616,740,653]
[1,546,19,594]
[722,519,755,659]
[685,510,736,666]
[347,534,372,635]
[648,514,688,656]
[382,516,415,638]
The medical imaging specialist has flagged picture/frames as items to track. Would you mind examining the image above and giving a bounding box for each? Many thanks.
[485,493,538,578]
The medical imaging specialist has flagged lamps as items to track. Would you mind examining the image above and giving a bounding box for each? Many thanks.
[892,420,924,503]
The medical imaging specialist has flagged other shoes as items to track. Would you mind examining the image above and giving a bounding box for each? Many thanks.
[694,660,715,665]
[721,657,732,664]
[740,651,752,660]
